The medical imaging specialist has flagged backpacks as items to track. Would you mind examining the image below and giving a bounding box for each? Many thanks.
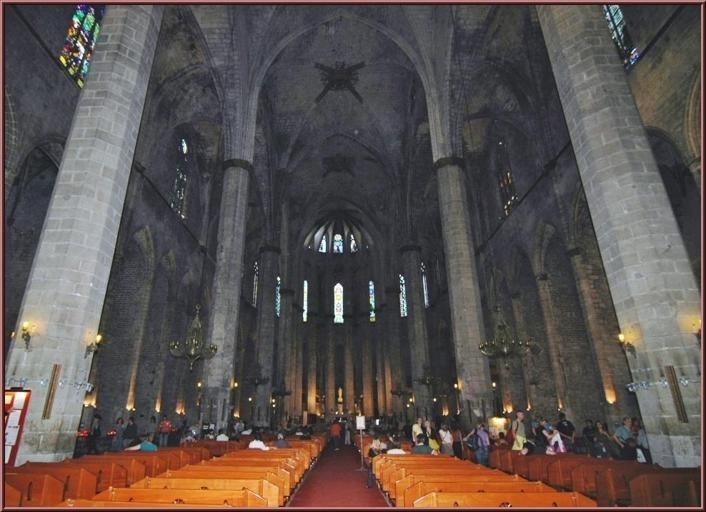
[467,428,486,452]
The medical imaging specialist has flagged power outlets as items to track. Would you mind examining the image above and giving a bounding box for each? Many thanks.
[616,333,636,357]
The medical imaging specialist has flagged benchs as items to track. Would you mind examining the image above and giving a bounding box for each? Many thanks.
[349,430,702,508]
[2,403,325,508]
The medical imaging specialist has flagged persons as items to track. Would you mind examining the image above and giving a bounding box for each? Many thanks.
[88,413,650,465]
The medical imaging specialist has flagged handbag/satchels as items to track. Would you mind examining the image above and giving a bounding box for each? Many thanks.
[506,420,519,444]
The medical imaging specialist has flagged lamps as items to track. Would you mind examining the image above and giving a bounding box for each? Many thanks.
[20,319,30,348]
[475,302,532,369]
[84,332,102,360]
[166,302,217,372]
[241,361,269,388]
[414,357,441,387]
